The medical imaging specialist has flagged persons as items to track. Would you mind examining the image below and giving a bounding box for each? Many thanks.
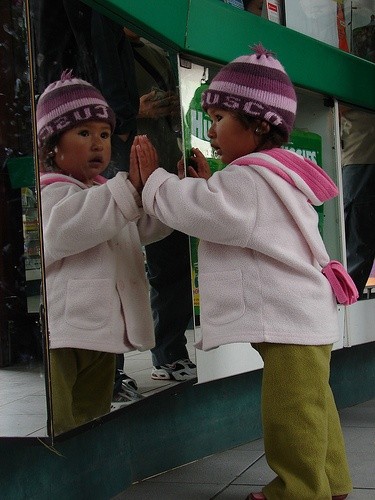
[41,0,197,435]
[338,101,375,299]
[136,43,359,500]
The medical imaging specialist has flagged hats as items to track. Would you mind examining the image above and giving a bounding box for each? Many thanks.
[202,44,297,142]
[37,70,114,157]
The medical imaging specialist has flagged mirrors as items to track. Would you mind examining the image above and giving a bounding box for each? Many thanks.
[0,0,48,438]
[28,0,197,437]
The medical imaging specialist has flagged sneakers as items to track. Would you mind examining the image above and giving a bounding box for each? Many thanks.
[150,359,196,381]
